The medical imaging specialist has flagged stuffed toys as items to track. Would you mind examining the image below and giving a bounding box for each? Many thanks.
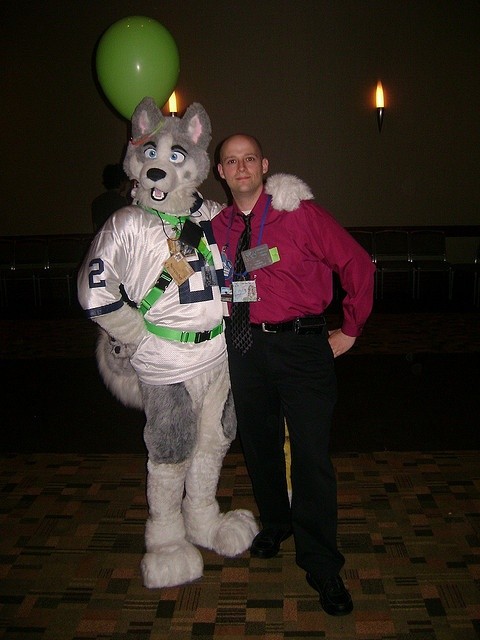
[76,97,314,588]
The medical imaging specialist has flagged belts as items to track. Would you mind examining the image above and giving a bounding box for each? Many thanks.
[251,322,294,333]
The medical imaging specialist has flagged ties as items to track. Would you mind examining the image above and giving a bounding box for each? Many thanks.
[228,214,254,359]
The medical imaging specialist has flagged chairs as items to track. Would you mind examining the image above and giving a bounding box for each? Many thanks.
[367,231,411,303]
[39,235,85,304]
[336,229,372,306]
[411,229,448,302]
[4,235,49,306]
[447,236,478,304]
[0,234,13,301]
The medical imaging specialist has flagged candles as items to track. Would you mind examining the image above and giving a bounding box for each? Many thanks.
[374,78,389,131]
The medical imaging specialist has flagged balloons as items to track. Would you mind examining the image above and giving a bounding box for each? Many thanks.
[95,16,180,122]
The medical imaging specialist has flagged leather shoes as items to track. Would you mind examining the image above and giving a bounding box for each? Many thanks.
[305,570,354,617]
[250,523,293,559]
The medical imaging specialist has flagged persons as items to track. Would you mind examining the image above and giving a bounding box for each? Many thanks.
[209,132,377,615]
